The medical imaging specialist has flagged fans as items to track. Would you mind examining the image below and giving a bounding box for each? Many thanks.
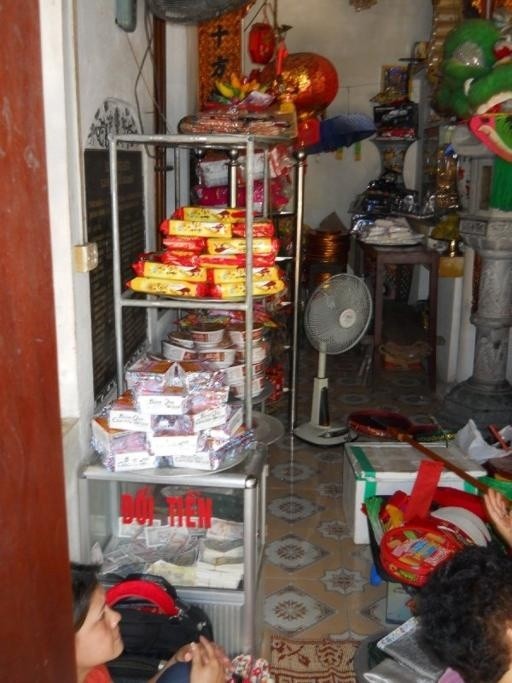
[295,271,372,451]
[115,0,248,32]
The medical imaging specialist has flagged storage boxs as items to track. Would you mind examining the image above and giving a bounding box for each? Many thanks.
[342,439,490,546]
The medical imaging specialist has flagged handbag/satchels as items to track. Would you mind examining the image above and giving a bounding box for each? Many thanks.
[106,574,213,683]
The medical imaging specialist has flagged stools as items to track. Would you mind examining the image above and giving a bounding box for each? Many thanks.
[358,235,440,374]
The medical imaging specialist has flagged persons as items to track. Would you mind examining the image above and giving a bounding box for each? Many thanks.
[68,559,236,683]
[409,486,512,683]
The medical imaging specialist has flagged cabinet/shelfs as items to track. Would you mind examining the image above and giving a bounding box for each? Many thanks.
[80,441,269,668]
